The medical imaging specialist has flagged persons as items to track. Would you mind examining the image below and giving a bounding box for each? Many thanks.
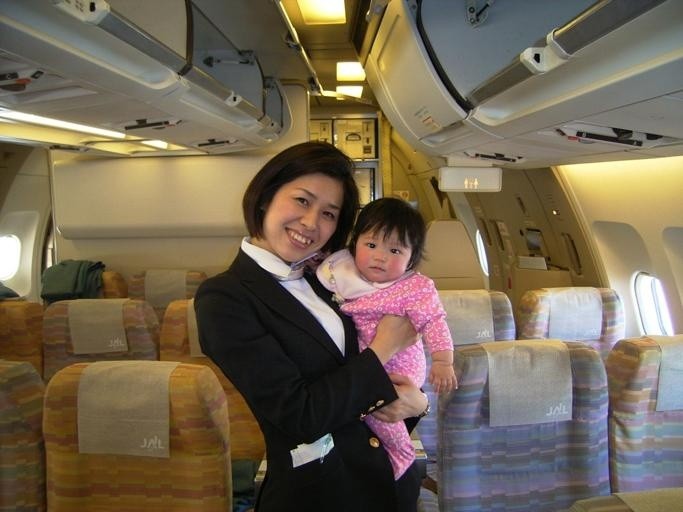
[193,138,423,511]
[315,194,460,483]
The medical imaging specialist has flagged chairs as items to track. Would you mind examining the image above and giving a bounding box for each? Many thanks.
[2,268,264,509]
[406,285,683,512]
